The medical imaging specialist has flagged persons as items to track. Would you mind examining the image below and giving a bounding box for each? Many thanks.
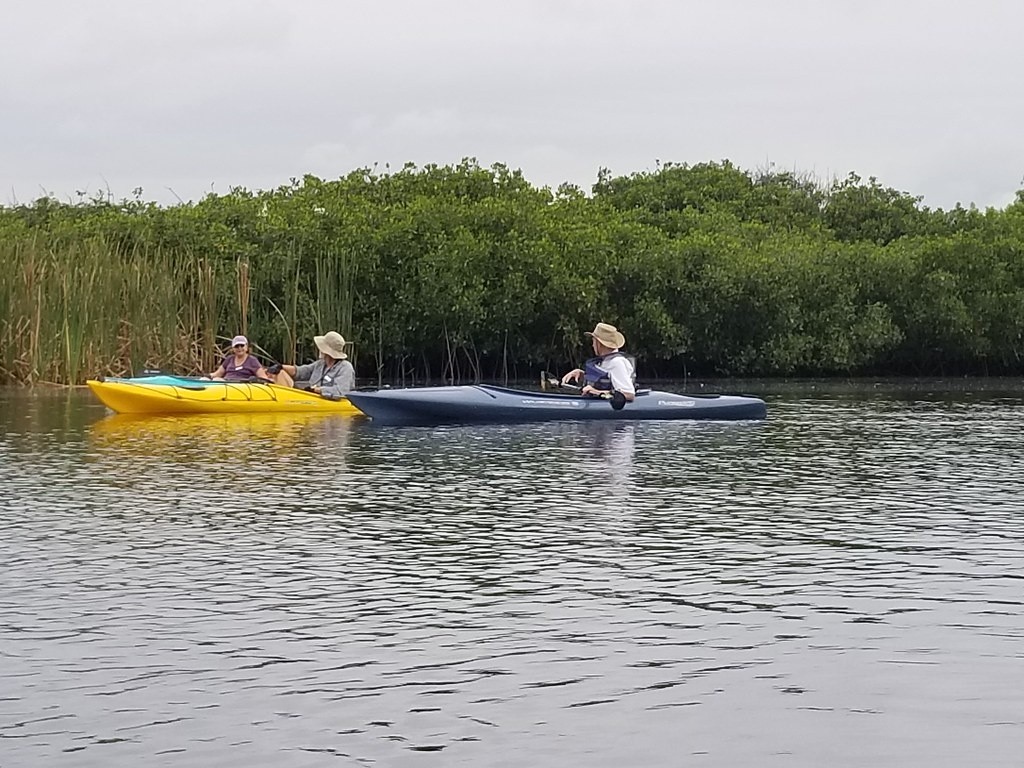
[199,335,268,383]
[563,324,636,402]
[268,332,356,402]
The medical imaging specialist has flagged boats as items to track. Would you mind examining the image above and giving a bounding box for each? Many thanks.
[70,373,370,415]
[338,381,771,428]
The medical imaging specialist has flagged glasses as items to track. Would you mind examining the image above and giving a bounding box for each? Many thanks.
[235,344,244,348]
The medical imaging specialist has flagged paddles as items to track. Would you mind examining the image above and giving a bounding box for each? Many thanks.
[540,369,626,411]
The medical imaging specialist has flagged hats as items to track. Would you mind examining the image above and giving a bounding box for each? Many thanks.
[584,322,625,349]
[313,331,348,360]
[231,335,248,348]
